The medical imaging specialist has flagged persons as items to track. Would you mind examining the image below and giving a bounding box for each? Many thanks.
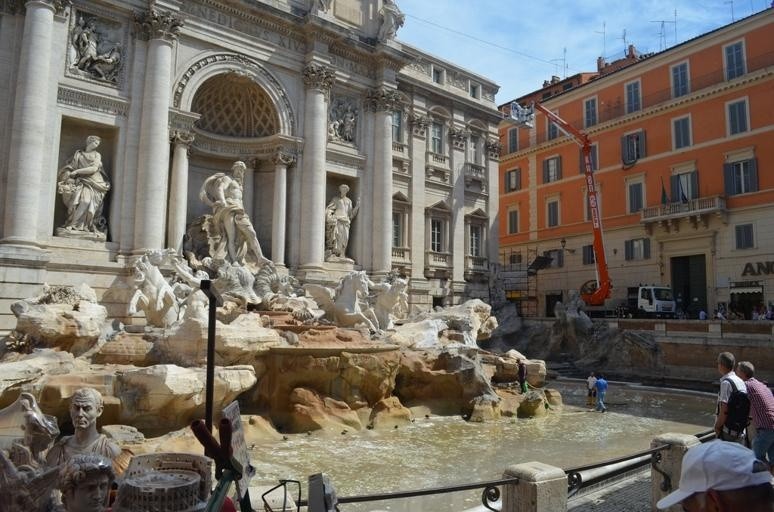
[69,14,122,83]
[56,453,114,511]
[585,370,598,408]
[43,386,122,468]
[325,182,363,260]
[713,351,750,449]
[676,300,774,321]
[198,158,277,271]
[515,357,527,395]
[552,288,593,328]
[376,1,407,45]
[55,134,112,241]
[365,273,409,331]
[168,256,210,320]
[328,96,356,144]
[594,373,608,412]
[734,360,774,468]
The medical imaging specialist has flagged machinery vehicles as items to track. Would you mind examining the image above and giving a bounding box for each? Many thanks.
[504,99,676,319]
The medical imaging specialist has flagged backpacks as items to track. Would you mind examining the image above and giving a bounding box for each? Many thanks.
[718,378,753,432]
